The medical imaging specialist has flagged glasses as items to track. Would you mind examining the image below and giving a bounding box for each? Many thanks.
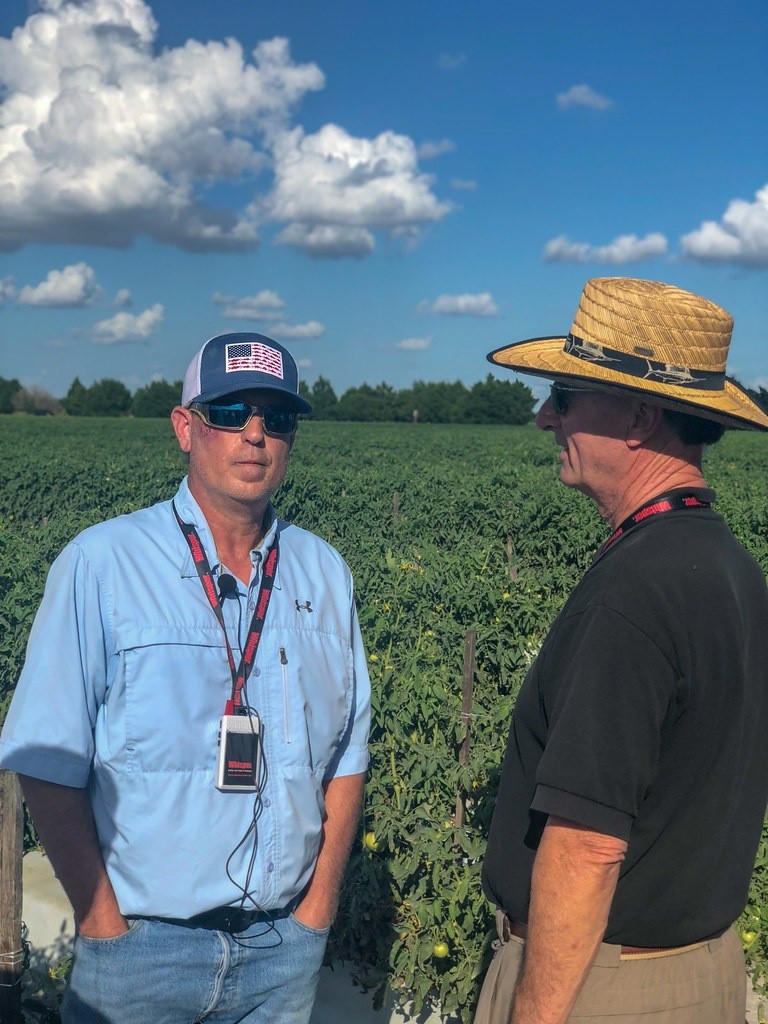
[549,384,621,417]
[188,397,300,438]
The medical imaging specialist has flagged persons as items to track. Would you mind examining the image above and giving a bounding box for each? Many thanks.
[472,276,768,1024]
[0,332,372,1024]
[215,408,242,426]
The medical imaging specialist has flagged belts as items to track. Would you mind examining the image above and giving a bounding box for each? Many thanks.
[123,897,304,933]
[506,917,683,954]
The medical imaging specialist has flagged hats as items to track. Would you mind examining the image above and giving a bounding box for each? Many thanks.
[486,277,768,432]
[181,331,313,414]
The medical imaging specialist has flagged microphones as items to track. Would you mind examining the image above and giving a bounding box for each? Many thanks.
[217,573,238,595]
